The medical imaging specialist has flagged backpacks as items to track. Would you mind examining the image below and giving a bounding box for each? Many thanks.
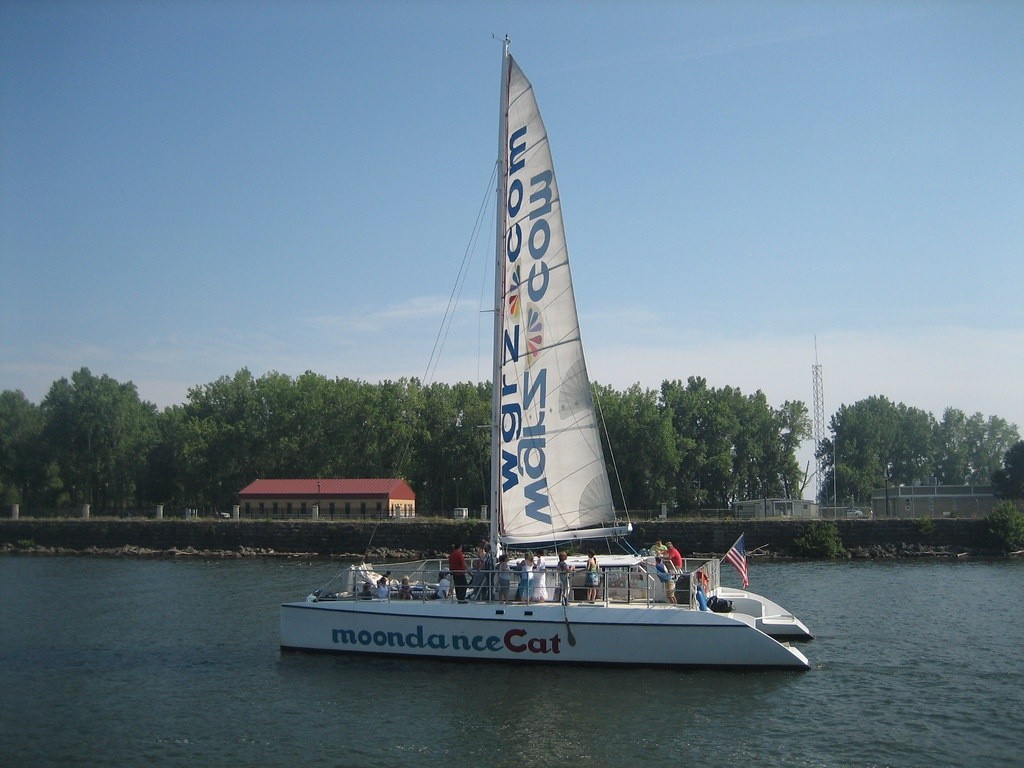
[707,596,736,613]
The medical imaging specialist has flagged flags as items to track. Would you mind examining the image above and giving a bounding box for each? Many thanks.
[726,534,748,589]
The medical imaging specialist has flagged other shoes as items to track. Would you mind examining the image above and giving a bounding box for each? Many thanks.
[457,599,467,604]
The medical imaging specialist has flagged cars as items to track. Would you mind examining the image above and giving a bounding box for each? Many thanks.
[847,510,863,516]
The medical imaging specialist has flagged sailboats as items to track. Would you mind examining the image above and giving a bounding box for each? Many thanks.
[280,31,817,671]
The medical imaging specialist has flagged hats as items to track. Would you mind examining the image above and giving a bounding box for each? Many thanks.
[666,542,673,546]
[441,568,448,571]
[402,575,408,579]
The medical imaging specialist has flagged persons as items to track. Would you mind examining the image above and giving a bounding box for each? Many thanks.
[650,557,678,603]
[374,571,391,598]
[517,550,548,603]
[587,549,599,600]
[425,567,451,600]
[478,530,512,603]
[557,552,574,606]
[650,540,668,554]
[398,576,414,600]
[361,583,372,600]
[449,543,473,603]
[656,542,682,579]
